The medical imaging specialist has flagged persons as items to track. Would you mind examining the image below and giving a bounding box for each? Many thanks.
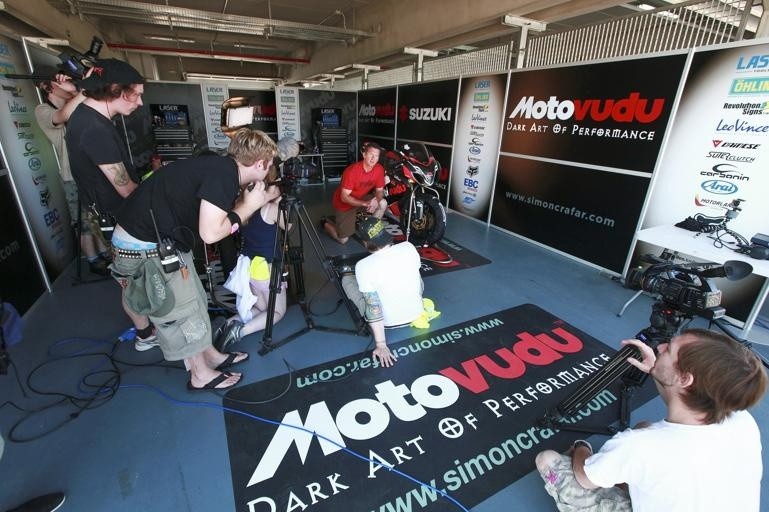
[32,55,160,353]
[315,142,388,245]
[536,327,768,512]
[341,215,424,369]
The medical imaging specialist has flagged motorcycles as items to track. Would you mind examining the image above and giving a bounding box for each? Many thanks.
[362,143,448,245]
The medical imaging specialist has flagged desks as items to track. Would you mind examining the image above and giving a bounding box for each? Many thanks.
[618,227,769,320]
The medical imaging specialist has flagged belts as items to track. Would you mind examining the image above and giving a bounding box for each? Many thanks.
[111,245,159,259]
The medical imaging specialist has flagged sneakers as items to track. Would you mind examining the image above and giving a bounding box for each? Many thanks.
[134,328,159,352]
[317,215,336,234]
[88,251,111,276]
[211,316,244,352]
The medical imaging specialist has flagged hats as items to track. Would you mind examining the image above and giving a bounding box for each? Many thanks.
[123,259,176,317]
[360,217,392,244]
[79,58,140,90]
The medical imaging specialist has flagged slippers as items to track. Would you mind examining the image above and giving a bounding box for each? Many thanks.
[187,352,249,391]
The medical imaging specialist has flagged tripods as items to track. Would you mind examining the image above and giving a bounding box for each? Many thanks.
[538,328,672,435]
[258,194,371,357]
